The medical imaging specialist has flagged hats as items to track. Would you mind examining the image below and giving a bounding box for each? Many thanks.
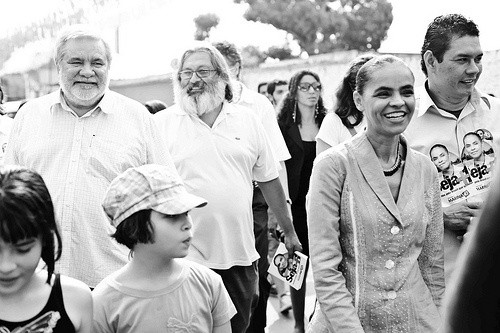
[101,164,207,228]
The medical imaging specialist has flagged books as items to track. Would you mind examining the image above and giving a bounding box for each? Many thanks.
[266,242,308,291]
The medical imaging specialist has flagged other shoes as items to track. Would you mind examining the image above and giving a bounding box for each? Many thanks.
[270,284,278,295]
[280,296,292,313]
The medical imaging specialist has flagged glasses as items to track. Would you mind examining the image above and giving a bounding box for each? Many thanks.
[297,82,320,92]
[179,67,217,79]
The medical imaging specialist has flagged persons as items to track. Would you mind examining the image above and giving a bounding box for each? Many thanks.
[314,53,380,157]
[91,164,237,333]
[6,23,152,292]
[430,129,496,207]
[274,252,297,277]
[212,40,292,333]
[401,12,500,287]
[151,43,302,333]
[305,54,446,333]
[277,70,327,333]
[0,166,93,333]
